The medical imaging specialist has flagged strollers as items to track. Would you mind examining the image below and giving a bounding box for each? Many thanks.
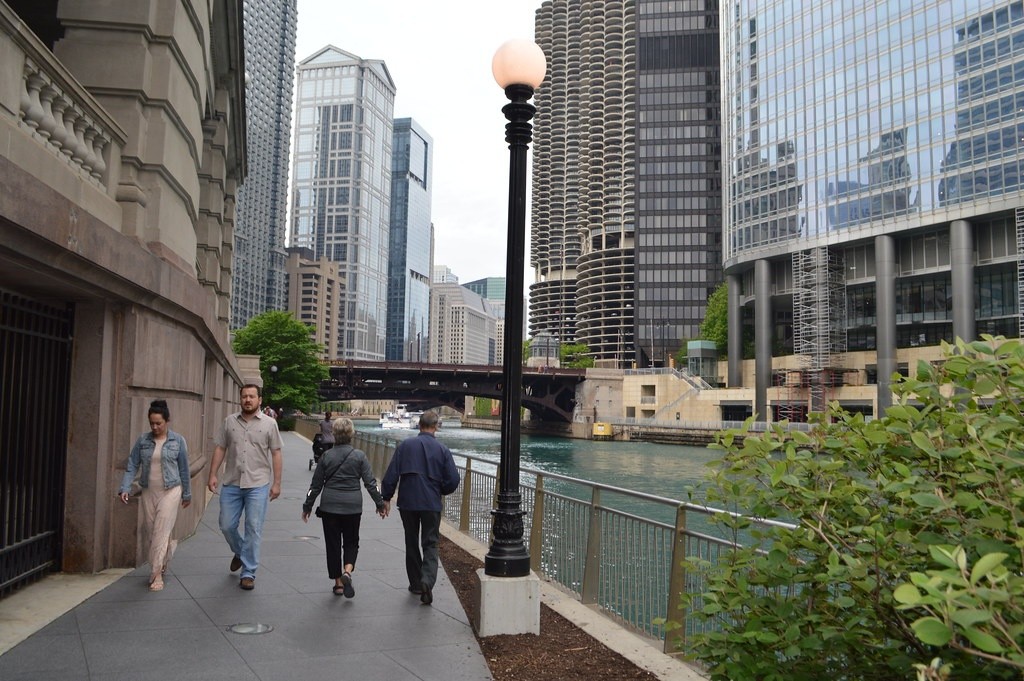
[309,434,332,471]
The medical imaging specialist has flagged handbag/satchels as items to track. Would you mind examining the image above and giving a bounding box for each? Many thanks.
[315,507,321,518]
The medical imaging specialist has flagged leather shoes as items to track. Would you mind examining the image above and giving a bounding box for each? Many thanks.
[230,554,242,571]
[241,578,254,588]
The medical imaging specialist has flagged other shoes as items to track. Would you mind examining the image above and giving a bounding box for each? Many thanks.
[162,561,167,574]
[149,581,164,591]
[420,581,433,603]
[409,585,422,593]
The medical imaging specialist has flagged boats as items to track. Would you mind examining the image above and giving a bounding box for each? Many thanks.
[379,405,419,431]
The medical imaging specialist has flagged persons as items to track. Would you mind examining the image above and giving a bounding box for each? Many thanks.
[261,404,277,418]
[301,418,388,598]
[208,383,285,591]
[376,411,461,604]
[319,411,335,452]
[120,399,192,591]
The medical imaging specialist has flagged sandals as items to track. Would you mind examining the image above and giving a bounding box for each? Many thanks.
[333,585,343,595]
[341,572,355,598]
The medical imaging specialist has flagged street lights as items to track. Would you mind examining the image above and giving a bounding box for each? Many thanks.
[485,39,547,578]
